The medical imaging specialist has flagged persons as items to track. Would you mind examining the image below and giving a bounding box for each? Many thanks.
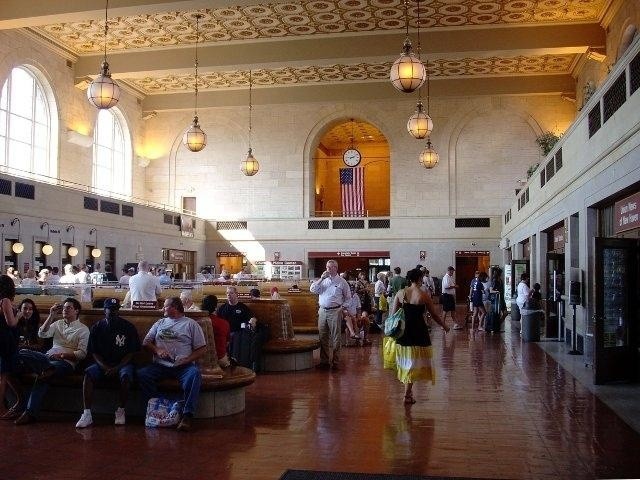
[117,261,171,309]
[201,285,258,364]
[1,274,208,430]
[1,263,104,291]
[440,267,462,328]
[516,274,532,337]
[309,257,376,370]
[203,270,280,299]
[469,267,508,334]
[372,262,450,406]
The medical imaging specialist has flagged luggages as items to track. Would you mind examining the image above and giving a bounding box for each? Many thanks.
[485,293,500,332]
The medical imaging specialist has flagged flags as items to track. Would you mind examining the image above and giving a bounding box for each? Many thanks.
[339,167,367,219]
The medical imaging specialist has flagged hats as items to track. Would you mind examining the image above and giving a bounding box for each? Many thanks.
[104,298,119,309]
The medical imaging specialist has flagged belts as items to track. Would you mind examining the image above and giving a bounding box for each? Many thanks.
[325,306,341,310]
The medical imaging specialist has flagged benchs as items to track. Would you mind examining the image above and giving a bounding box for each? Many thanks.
[0,279,320,419]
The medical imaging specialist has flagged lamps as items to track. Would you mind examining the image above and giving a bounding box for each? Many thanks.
[11,218,102,258]
[240,69,260,176]
[390,0,439,170]
[87,0,121,108]
[184,17,207,152]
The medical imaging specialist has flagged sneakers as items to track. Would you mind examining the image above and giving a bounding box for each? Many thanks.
[76,414,92,428]
[4,408,35,424]
[454,324,463,330]
[114,408,125,424]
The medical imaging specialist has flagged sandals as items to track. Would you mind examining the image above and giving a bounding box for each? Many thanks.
[404,396,416,404]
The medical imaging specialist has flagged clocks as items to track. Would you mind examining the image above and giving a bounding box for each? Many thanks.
[343,149,361,166]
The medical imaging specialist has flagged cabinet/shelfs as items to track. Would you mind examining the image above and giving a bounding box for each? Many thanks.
[257,265,302,279]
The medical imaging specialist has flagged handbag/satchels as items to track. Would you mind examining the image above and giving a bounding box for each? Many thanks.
[384,308,405,339]
[145,397,184,427]
